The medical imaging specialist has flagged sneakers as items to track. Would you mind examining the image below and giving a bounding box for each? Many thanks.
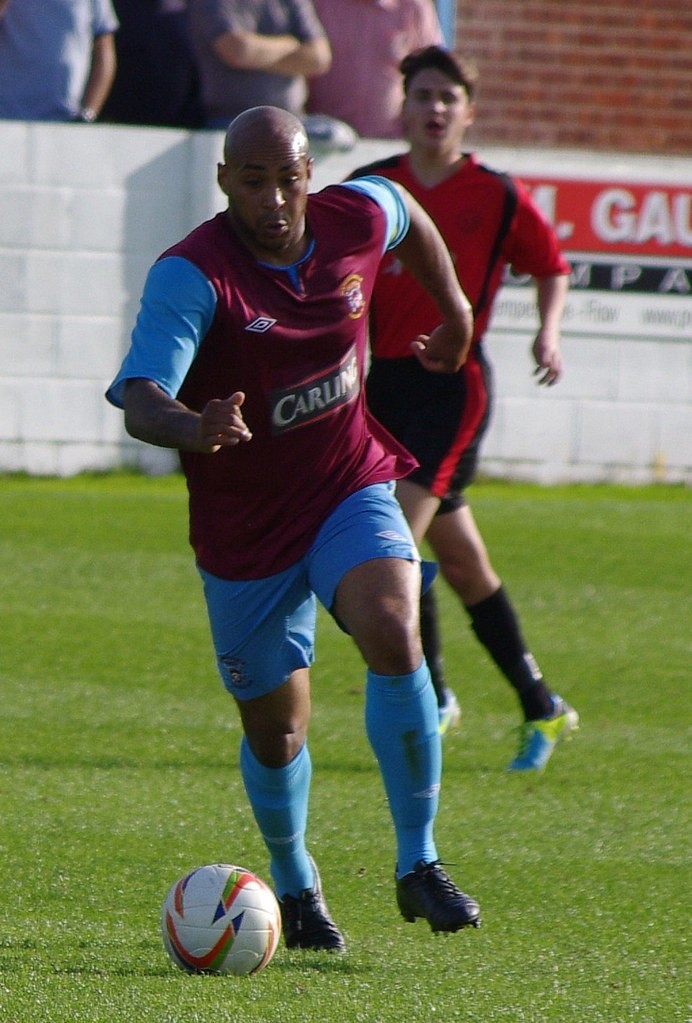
[434,689,460,735]
[277,851,346,954]
[506,692,579,770]
[392,859,481,933]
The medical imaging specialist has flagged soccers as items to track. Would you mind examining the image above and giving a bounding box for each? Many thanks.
[161,863,284,975]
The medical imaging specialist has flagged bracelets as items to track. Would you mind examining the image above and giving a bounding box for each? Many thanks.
[80,105,98,125]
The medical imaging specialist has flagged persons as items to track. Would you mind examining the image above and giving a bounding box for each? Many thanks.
[105,105,484,950]
[331,43,578,780]
[0,0,446,138]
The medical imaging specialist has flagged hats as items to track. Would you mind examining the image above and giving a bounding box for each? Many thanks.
[401,44,476,100]
